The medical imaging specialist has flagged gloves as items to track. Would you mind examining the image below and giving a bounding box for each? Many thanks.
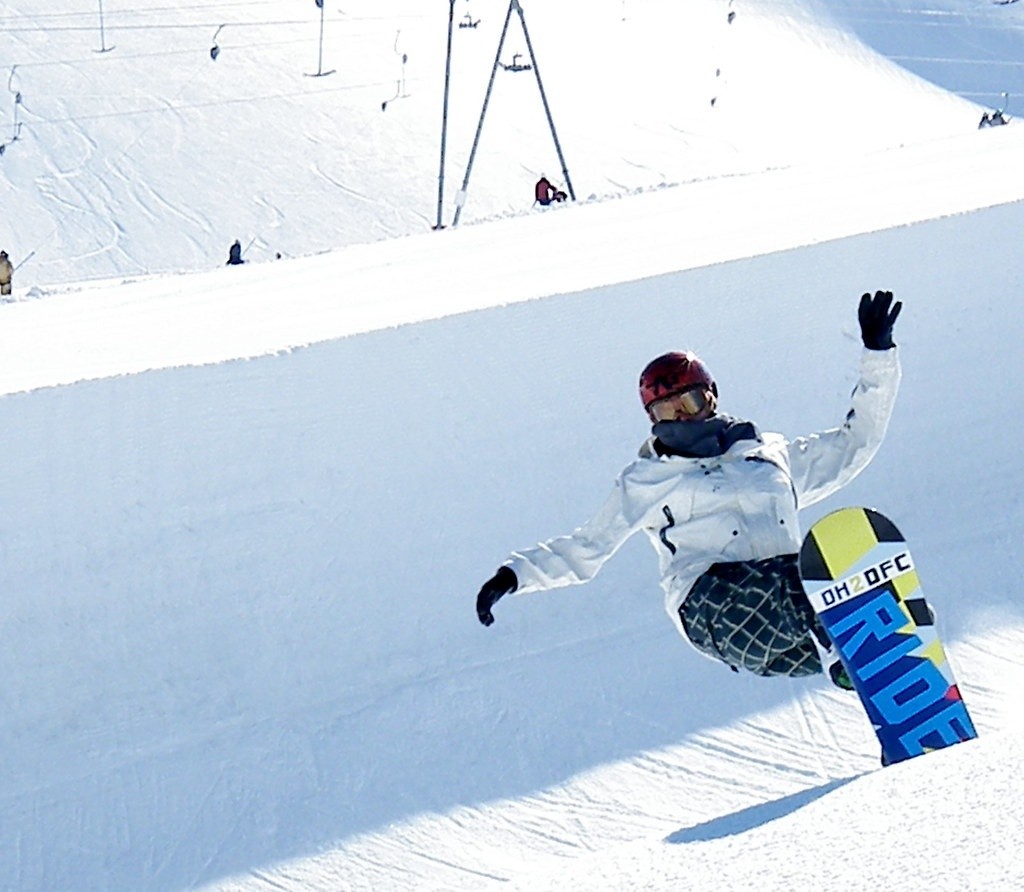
[475,567,517,626]
[858,290,902,351]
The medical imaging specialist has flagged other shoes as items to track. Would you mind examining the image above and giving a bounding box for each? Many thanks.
[822,604,937,692]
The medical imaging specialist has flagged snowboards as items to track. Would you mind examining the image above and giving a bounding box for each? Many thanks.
[797,506,979,763]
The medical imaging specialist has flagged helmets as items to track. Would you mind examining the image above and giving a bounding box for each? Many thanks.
[639,352,718,411]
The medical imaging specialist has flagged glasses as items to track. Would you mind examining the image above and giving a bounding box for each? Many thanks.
[649,389,710,425]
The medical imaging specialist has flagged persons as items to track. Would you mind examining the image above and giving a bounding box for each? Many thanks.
[477,292,935,691]
[230,240,244,264]
[536,177,567,205]
[978,109,1008,129]
[0,251,14,294]
